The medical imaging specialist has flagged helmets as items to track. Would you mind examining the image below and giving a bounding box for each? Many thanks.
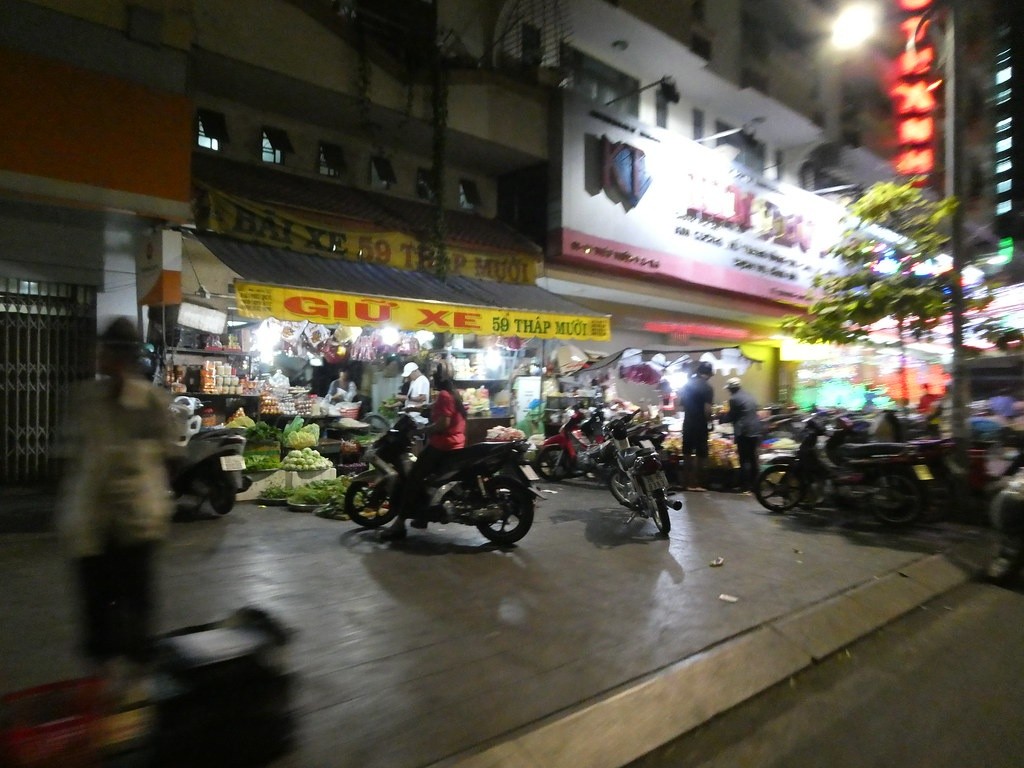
[431,362,455,385]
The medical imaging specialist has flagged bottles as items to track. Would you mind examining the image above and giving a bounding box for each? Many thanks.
[206,335,222,350]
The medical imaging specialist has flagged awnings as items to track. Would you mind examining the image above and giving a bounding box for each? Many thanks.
[185,228,612,340]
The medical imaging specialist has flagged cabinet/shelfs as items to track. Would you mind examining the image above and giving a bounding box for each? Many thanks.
[147,305,261,423]
[426,347,516,444]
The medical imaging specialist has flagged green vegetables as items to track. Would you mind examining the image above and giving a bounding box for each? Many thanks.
[353,432,376,444]
[242,421,283,443]
[258,472,381,516]
[241,454,282,471]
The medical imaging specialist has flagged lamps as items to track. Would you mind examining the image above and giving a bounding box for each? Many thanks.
[605,75,680,107]
[694,117,767,142]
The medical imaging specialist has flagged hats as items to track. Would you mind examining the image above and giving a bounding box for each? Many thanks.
[722,377,742,390]
[696,361,713,376]
[401,361,419,377]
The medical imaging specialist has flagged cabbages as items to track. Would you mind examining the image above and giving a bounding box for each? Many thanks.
[281,446,334,471]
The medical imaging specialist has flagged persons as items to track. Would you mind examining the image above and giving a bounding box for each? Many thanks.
[59,316,189,669]
[678,361,715,491]
[328,362,466,539]
[717,377,762,495]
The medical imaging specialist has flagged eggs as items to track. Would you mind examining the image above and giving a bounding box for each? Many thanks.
[278,400,312,415]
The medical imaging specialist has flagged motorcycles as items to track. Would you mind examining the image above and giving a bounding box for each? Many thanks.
[531,399,677,488]
[346,409,546,545]
[597,408,683,537]
[755,412,944,527]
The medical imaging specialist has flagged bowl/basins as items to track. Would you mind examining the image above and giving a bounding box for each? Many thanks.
[289,502,326,511]
[257,496,287,505]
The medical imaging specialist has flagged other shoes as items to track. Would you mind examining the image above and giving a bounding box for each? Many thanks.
[380,527,407,538]
[687,486,707,491]
[410,520,427,529]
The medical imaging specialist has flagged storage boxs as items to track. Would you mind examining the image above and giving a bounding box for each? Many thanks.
[243,441,281,458]
[236,470,286,502]
[286,468,336,487]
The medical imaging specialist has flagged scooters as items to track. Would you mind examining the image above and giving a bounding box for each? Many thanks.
[163,397,255,516]
